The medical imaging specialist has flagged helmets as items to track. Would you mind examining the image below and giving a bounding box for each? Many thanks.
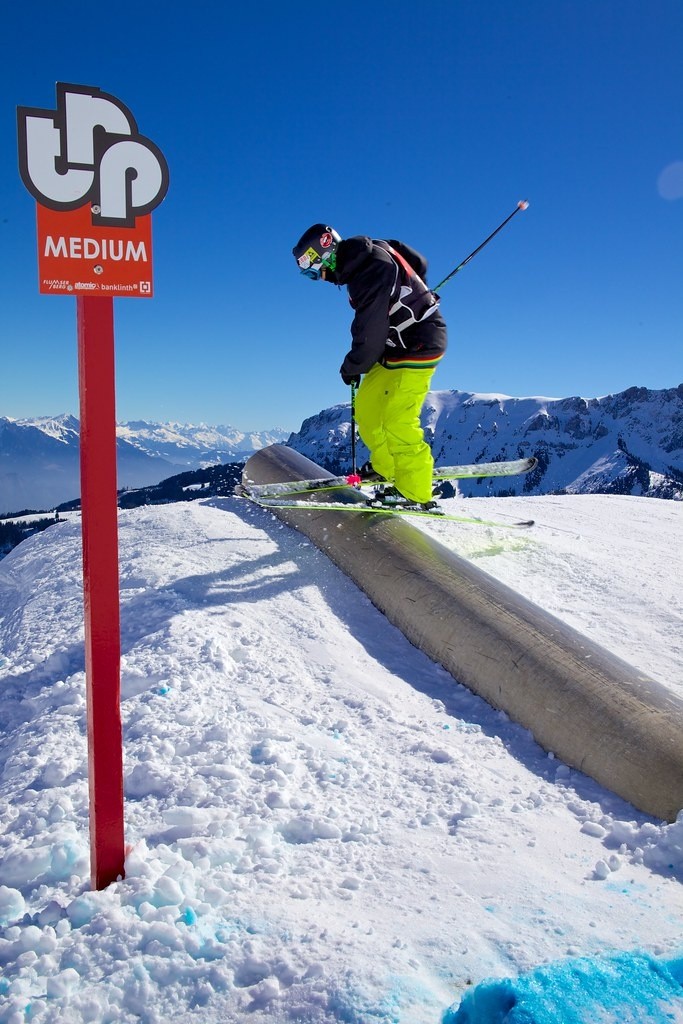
[292,222,342,273]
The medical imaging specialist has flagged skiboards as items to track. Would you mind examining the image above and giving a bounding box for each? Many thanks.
[239,492,535,530]
[234,456,539,498]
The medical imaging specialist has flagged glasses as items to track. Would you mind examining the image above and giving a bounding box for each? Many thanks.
[301,268,320,280]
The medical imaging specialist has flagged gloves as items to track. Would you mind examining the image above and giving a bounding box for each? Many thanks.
[339,370,362,390]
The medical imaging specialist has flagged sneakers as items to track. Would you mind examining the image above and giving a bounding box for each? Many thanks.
[366,484,428,511]
[357,460,387,484]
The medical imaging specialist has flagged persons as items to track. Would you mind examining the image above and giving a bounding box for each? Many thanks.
[292,223,447,509]
